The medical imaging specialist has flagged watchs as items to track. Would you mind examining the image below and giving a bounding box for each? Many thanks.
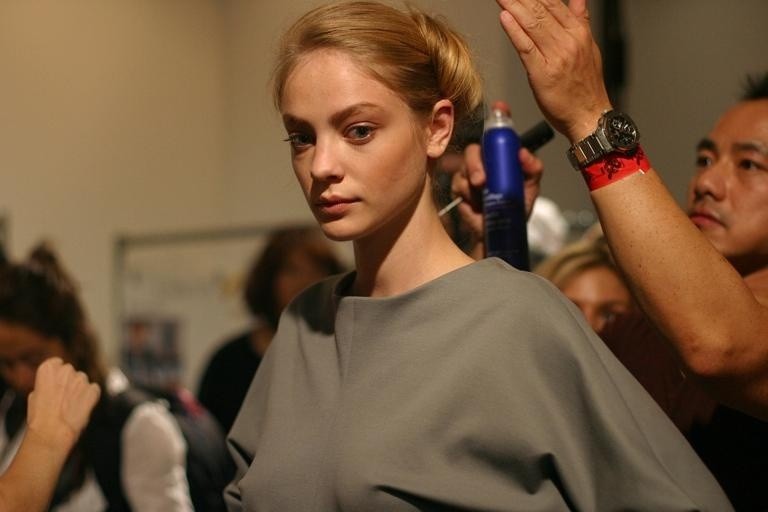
[570,108,646,171]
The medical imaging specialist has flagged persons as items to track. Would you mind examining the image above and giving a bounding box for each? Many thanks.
[1,356,100,510]
[198,220,343,434]
[221,1,741,512]
[0,238,233,511]
[451,1,768,432]
[431,151,640,335]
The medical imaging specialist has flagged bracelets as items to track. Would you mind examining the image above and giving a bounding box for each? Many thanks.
[583,147,650,192]
